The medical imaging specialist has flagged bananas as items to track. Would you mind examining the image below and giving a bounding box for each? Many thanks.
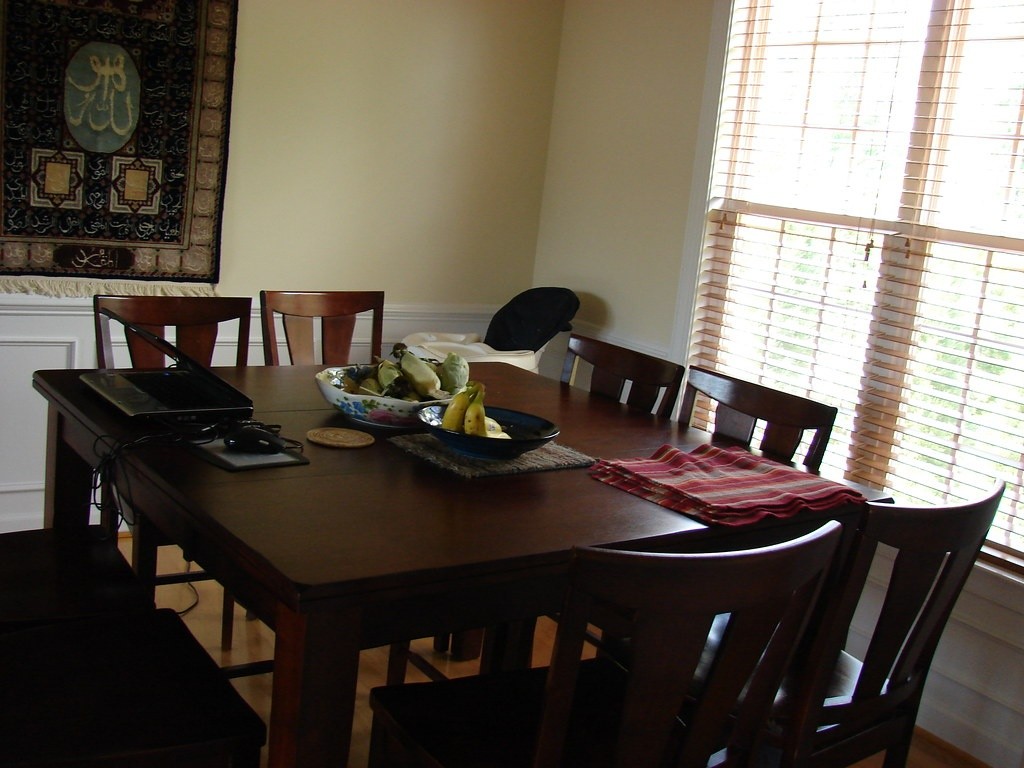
[442,380,512,439]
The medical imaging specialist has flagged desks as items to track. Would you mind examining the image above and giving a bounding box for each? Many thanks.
[28,355,895,768]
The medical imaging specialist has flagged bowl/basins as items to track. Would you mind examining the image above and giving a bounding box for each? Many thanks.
[418,404,561,463]
[315,364,452,428]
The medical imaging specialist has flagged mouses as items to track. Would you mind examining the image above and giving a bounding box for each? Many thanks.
[224,427,287,455]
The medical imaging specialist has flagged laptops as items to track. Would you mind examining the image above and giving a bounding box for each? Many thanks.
[79,307,254,432]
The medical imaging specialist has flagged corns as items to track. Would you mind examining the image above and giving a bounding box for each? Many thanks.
[358,342,471,402]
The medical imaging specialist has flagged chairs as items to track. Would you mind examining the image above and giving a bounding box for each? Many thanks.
[362,517,843,768]
[247,290,453,621]
[381,334,686,687]
[583,365,836,650]
[94,294,252,653]
[0,524,156,630]
[598,490,1009,768]
[0,607,269,768]
